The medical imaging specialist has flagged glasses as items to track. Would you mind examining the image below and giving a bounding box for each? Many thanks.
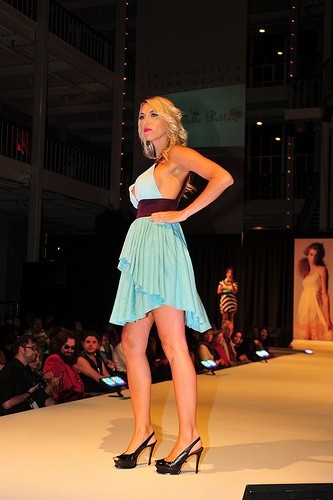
[24,345,40,351]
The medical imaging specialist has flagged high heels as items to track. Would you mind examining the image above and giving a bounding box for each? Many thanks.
[114,430,157,469]
[154,436,204,475]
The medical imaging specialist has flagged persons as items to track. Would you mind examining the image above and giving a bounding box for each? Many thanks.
[298,242,333,341]
[109,97,234,474]
[217,268,237,329]
[0,313,266,417]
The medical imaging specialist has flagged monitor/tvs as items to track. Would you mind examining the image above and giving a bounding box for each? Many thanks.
[98,376,128,397]
[200,360,218,375]
[256,350,270,363]
